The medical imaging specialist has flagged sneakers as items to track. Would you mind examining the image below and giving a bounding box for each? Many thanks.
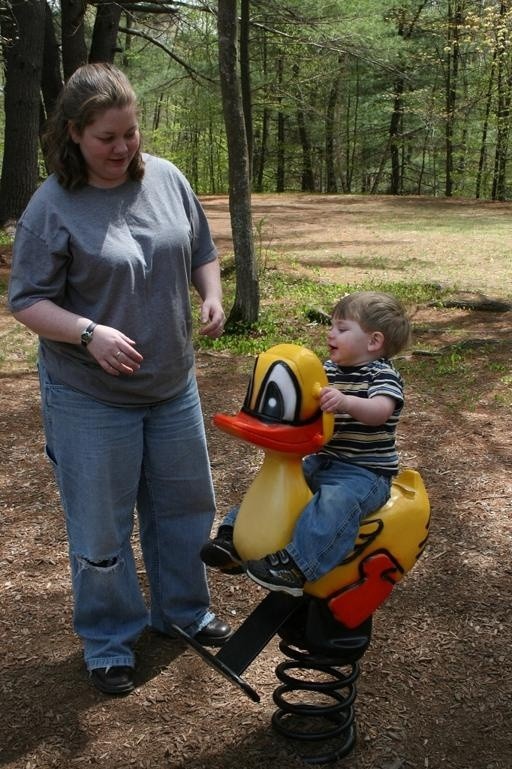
[200,526,251,575]
[244,553,306,601]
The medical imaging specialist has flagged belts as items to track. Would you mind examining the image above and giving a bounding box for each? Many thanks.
[190,616,230,641]
[91,665,133,695]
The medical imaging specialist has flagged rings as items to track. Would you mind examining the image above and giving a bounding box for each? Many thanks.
[115,351,122,358]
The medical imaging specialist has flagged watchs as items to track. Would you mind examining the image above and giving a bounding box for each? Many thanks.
[81,321,96,346]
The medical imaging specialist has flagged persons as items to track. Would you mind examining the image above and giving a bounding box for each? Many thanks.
[199,289,407,599]
[8,63,232,693]
